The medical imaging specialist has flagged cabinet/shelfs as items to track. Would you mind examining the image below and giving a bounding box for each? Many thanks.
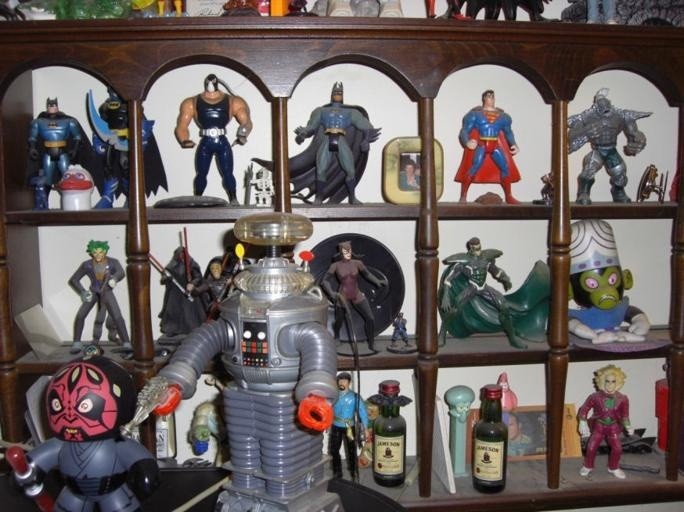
[0,18,683,512]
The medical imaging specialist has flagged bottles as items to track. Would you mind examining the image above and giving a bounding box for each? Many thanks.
[472,385,508,492]
[156,410,178,459]
[374,379,406,487]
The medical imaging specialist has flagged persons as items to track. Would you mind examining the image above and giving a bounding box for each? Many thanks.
[16,0,646,512]
[0,2,684,510]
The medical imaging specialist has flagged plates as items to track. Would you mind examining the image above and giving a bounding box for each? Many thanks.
[300,233,404,343]
